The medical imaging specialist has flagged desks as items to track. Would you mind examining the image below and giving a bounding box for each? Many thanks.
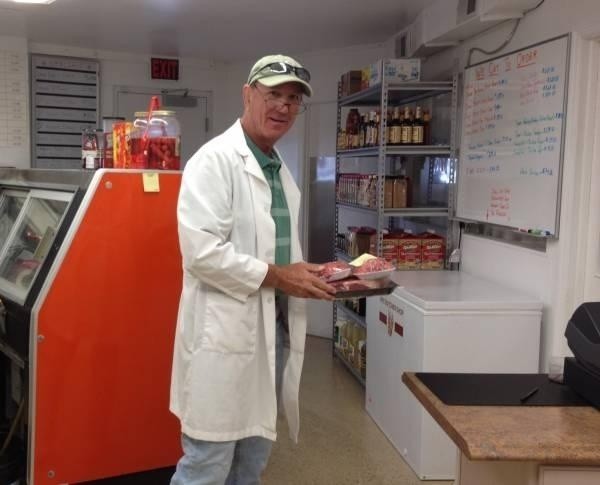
[400,371,599,484]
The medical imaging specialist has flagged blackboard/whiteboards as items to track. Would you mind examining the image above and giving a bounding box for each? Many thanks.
[449,31,572,240]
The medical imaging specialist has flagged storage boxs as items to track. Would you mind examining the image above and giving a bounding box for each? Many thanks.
[344,232,376,258]
[341,57,421,97]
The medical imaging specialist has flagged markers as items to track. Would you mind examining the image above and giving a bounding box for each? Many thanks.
[521,228,550,235]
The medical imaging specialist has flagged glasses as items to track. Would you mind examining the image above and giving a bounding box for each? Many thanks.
[254,85,306,114]
[248,63,310,85]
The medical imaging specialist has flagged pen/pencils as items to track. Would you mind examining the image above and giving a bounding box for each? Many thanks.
[520,387,540,401]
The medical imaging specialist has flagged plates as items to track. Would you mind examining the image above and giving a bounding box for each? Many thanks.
[323,267,353,282]
[355,266,396,280]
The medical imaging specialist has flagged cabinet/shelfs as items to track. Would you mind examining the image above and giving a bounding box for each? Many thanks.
[331,57,461,390]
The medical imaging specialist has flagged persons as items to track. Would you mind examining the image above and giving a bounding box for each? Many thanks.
[168,54,337,484]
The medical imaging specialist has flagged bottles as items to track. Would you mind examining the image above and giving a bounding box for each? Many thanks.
[147,110,182,170]
[346,225,377,259]
[81,127,105,169]
[334,316,366,373]
[128,110,147,169]
[337,105,432,147]
[335,173,410,210]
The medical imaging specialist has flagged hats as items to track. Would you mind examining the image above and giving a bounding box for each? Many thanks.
[246,54,313,98]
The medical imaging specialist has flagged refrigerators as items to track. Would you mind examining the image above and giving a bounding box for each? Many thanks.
[364,268,546,482]
